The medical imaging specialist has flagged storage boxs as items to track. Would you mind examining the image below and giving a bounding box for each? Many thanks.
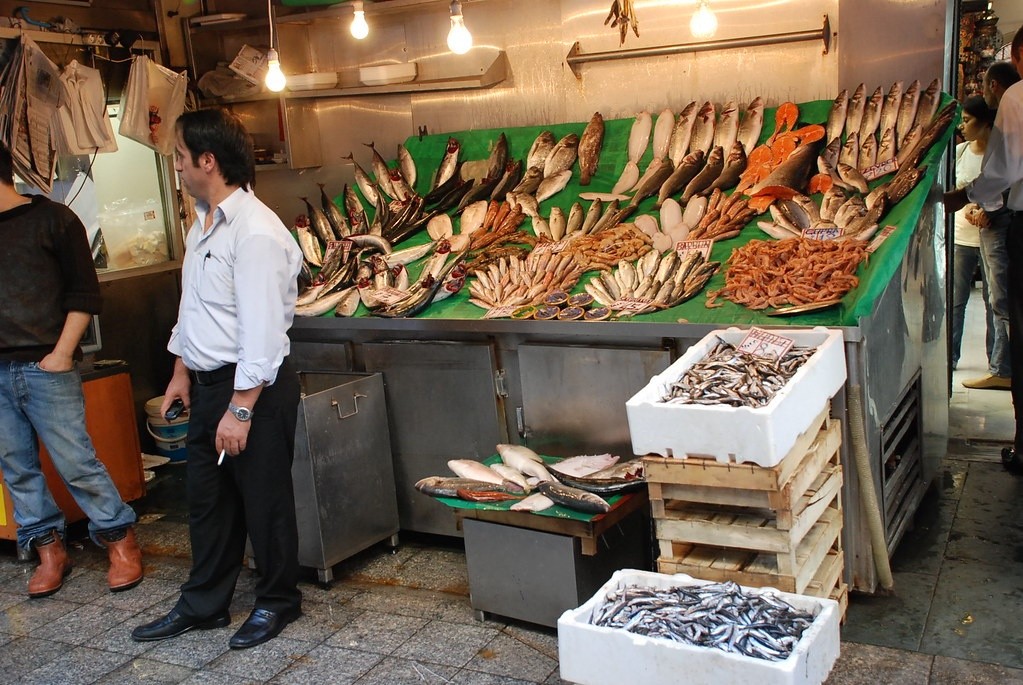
[556,568,839,685]
[627,327,847,465]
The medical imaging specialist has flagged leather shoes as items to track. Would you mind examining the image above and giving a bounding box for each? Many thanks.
[132,602,231,641]
[229,606,301,647]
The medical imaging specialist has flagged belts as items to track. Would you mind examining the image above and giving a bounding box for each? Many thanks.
[190,364,237,386]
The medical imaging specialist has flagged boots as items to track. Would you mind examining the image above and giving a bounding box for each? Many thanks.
[28,527,71,598]
[94,525,143,591]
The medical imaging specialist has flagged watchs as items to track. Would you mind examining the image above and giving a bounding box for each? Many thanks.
[228,401,254,421]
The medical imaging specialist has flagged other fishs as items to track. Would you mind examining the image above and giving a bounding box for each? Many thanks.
[588,579,816,662]
[291,79,960,323]
[414,443,645,514]
[654,334,817,410]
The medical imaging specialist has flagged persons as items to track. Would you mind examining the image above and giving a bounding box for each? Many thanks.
[0,137,142,596]
[952,26,1023,391]
[131,107,303,650]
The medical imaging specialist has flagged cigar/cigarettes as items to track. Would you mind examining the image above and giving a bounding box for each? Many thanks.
[218,449,225,466]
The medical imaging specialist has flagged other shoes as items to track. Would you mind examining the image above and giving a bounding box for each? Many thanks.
[961,372,1012,390]
[952,361,957,370]
[1000,447,1023,478]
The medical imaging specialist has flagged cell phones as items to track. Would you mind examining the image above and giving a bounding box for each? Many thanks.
[164,401,186,420]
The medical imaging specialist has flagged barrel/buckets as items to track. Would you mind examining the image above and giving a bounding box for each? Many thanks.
[145,396,191,464]
